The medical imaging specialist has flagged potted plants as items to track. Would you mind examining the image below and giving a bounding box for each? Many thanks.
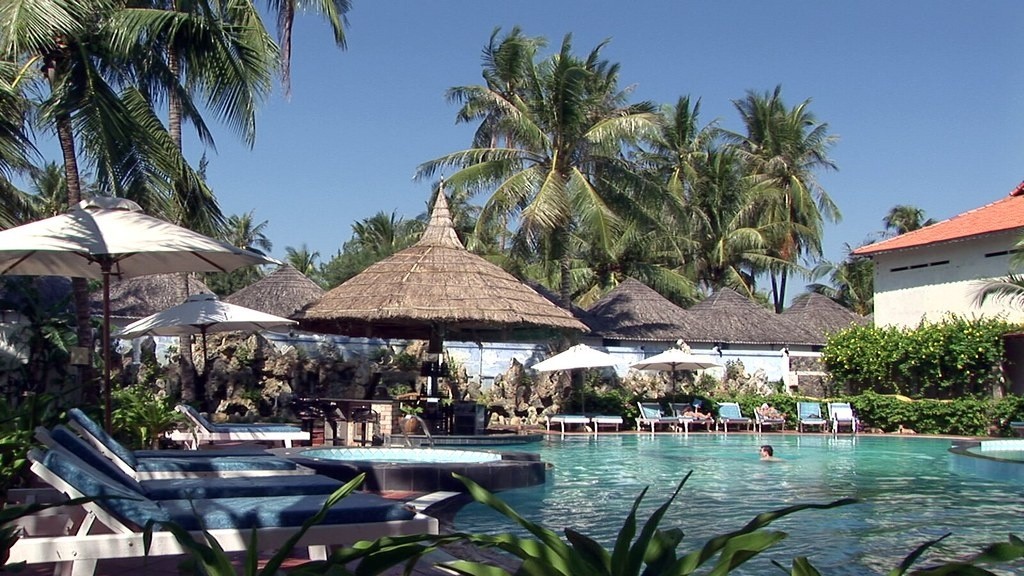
[109,390,199,451]
[396,404,424,436]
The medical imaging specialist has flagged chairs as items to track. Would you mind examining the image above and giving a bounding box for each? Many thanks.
[0,401,439,576]
[541,414,590,432]
[577,412,623,432]
[796,401,827,433]
[634,402,681,433]
[752,406,785,432]
[826,401,857,434]
[668,402,714,433]
[715,401,751,432]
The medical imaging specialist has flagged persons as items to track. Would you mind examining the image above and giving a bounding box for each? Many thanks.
[681,406,711,419]
[758,403,787,419]
[759,445,774,457]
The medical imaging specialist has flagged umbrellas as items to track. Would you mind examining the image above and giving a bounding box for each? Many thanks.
[531,343,628,426]
[0,197,285,435]
[114,291,300,443]
[630,347,724,428]
